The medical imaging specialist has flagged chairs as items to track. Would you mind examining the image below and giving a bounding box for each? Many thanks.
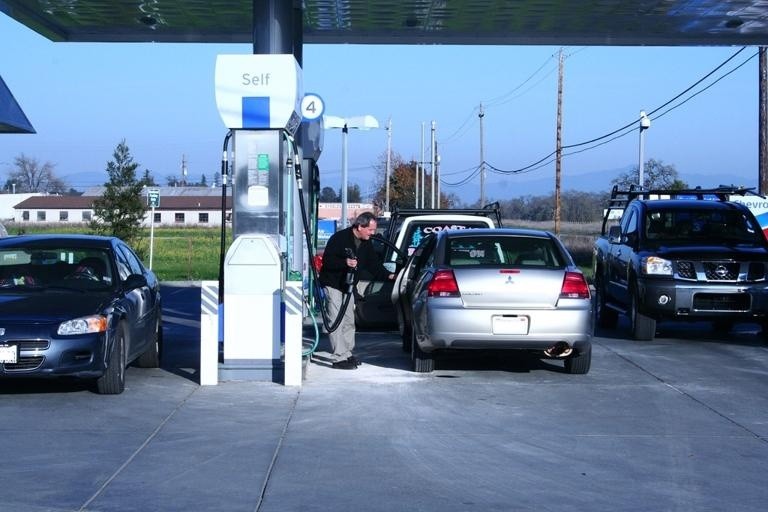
[648,218,704,239]
[449,248,546,266]
[78,258,107,280]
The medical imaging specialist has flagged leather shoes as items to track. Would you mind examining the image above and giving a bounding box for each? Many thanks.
[332,356,361,369]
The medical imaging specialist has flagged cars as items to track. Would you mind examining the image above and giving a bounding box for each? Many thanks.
[0,233,164,396]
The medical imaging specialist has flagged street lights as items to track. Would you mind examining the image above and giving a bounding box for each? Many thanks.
[637,109,651,201]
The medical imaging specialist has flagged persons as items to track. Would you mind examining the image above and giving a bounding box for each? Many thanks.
[317,212,379,370]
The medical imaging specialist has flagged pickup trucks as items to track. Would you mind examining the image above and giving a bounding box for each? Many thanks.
[592,182,768,343]
